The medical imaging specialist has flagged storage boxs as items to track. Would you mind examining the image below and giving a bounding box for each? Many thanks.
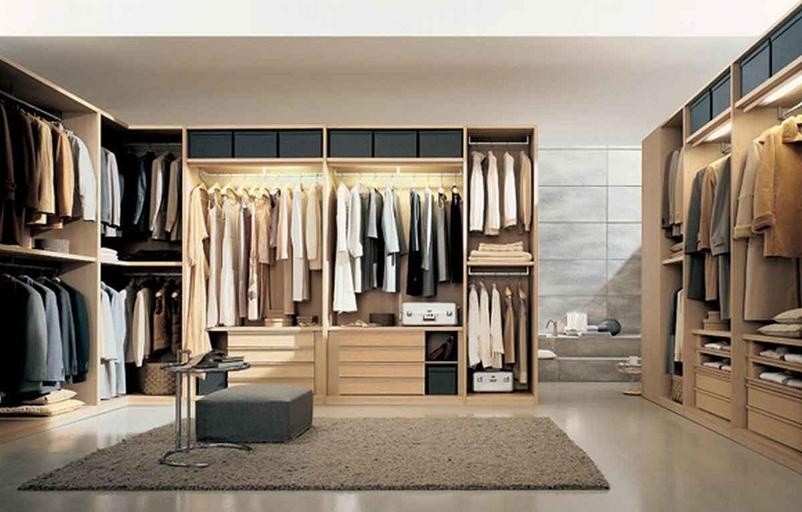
[187,130,234,158]
[374,130,417,157]
[279,130,324,157]
[417,128,464,158]
[471,371,515,393]
[328,130,373,158]
[428,366,458,396]
[233,129,278,157]
[400,301,461,328]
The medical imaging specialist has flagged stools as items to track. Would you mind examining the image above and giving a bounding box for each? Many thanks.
[193,383,315,440]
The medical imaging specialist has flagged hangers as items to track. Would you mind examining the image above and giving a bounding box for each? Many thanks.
[663,143,683,167]
[741,108,802,157]
[687,146,731,192]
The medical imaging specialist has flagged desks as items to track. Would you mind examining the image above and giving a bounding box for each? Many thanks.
[160,360,252,471]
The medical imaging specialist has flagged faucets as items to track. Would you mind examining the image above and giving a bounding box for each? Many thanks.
[546,318,557,336]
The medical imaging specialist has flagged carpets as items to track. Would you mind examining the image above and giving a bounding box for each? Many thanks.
[17,415,611,490]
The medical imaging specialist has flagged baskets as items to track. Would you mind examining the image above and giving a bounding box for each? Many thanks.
[672,375,683,402]
[139,362,176,395]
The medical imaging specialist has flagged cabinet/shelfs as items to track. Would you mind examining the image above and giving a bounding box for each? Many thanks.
[466,125,541,407]
[185,125,324,408]
[641,2,802,477]
[4,55,99,448]
[100,107,184,414]
[326,126,464,406]
